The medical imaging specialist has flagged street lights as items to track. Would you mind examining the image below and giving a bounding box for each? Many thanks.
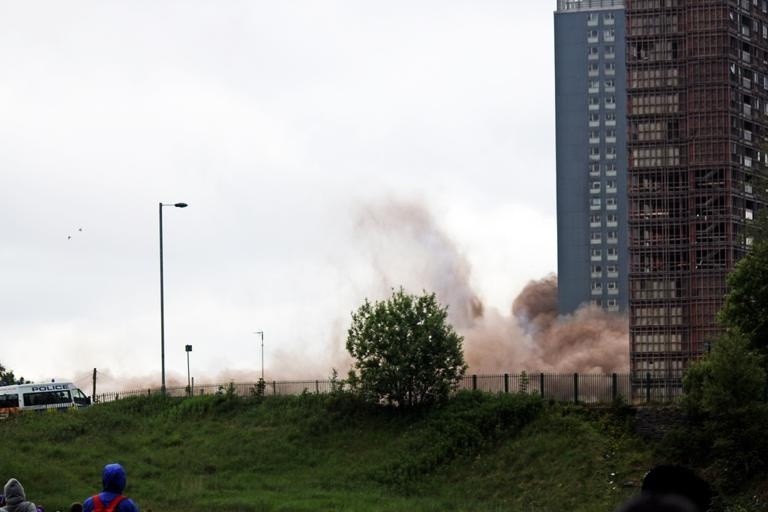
[253,329,267,397]
[157,200,197,398]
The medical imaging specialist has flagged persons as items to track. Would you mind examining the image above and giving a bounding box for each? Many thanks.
[0,478,37,512]
[70,502,82,512]
[218,387,226,394]
[83,463,137,512]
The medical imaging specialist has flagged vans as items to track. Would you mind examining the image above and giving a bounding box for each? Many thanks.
[1,379,94,419]
